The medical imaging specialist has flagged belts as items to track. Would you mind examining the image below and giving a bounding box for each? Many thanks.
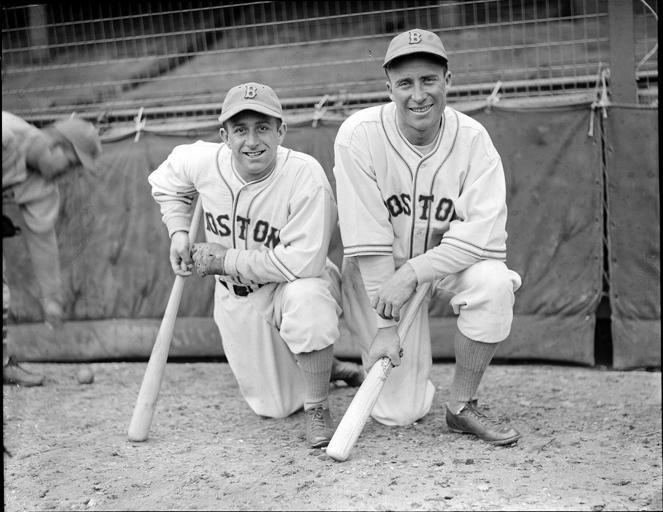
[218,277,273,296]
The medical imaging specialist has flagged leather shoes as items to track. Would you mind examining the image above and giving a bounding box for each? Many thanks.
[302,407,335,448]
[446,402,524,446]
[329,359,366,386]
[2,359,45,388]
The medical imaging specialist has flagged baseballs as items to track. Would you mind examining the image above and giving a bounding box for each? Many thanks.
[77,369,93,383]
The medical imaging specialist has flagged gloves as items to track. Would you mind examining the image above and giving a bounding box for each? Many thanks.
[192,242,229,276]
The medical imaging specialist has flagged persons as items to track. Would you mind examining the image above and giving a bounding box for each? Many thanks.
[0,107,106,388]
[145,81,365,450]
[331,27,524,449]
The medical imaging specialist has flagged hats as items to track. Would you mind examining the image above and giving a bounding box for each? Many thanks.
[381,27,448,67]
[218,82,281,124]
[51,116,103,173]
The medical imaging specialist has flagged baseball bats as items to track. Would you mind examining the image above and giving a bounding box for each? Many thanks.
[325,279,430,462]
[128,197,202,441]
